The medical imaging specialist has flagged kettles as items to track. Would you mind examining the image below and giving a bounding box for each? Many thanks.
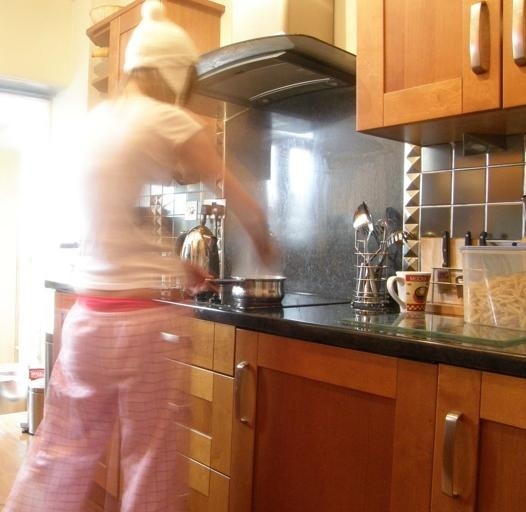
[174,216,219,290]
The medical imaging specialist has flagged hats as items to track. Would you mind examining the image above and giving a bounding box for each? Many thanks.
[123,2,199,97]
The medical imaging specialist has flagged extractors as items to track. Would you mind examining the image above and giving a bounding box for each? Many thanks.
[192,0,355,112]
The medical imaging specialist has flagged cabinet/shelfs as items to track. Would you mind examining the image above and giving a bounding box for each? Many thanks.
[354,0,526,145]
[430,363,526,512]
[53,292,238,512]
[228,327,437,512]
[85,0,225,185]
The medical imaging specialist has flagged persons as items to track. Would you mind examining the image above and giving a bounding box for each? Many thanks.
[3,0,282,511]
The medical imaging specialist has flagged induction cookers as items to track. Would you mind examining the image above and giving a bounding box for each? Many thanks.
[199,292,352,310]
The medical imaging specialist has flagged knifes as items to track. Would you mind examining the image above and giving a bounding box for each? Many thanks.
[439,231,453,293]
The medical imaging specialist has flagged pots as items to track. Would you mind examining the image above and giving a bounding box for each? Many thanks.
[203,274,285,304]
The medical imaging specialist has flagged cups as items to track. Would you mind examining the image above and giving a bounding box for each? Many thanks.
[387,271,430,314]
